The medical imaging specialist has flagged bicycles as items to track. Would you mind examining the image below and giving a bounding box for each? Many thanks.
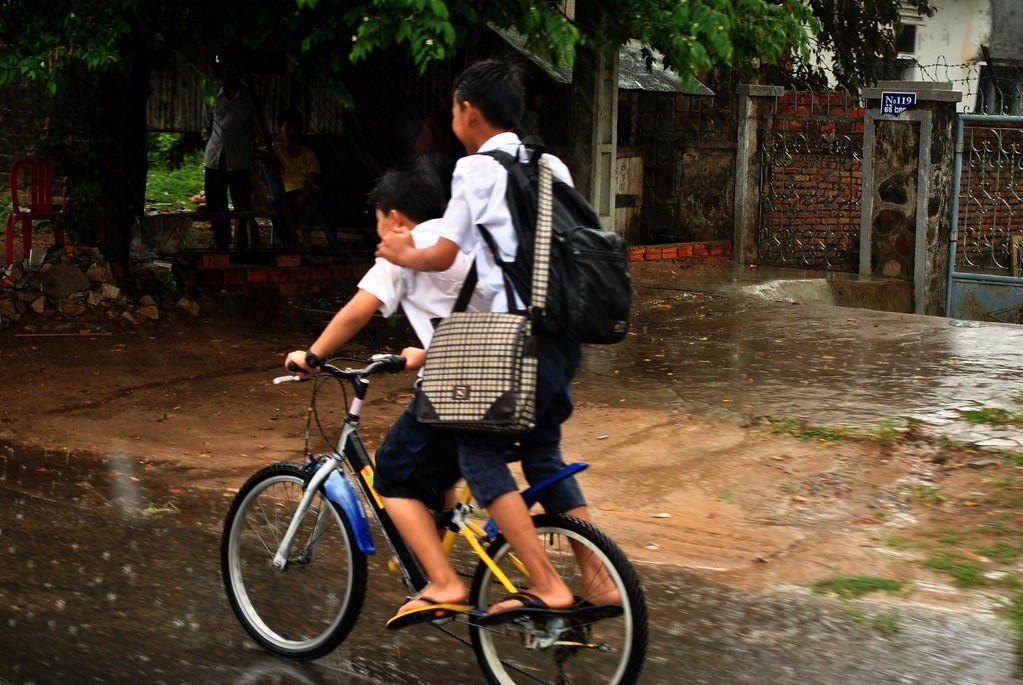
[219,351,650,685]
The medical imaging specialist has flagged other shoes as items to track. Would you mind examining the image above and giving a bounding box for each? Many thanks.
[328,244,351,256]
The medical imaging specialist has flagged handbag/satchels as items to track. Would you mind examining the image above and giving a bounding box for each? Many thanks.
[415,223,535,430]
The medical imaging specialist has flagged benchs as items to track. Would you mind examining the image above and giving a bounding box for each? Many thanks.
[186,209,265,252]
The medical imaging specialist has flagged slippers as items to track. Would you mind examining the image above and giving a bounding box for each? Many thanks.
[478,592,570,624]
[570,596,623,621]
[384,596,475,629]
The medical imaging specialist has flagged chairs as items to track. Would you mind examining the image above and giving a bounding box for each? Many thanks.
[5,156,65,267]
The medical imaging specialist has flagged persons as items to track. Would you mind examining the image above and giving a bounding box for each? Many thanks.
[373,56,625,626]
[286,167,503,631]
[199,60,259,252]
[250,111,339,248]
[415,72,466,217]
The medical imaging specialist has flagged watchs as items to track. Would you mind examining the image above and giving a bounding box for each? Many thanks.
[305,347,327,369]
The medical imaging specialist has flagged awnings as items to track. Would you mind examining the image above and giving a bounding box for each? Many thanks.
[483,21,714,97]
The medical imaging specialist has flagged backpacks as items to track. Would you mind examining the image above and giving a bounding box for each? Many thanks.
[475,136,630,344]
